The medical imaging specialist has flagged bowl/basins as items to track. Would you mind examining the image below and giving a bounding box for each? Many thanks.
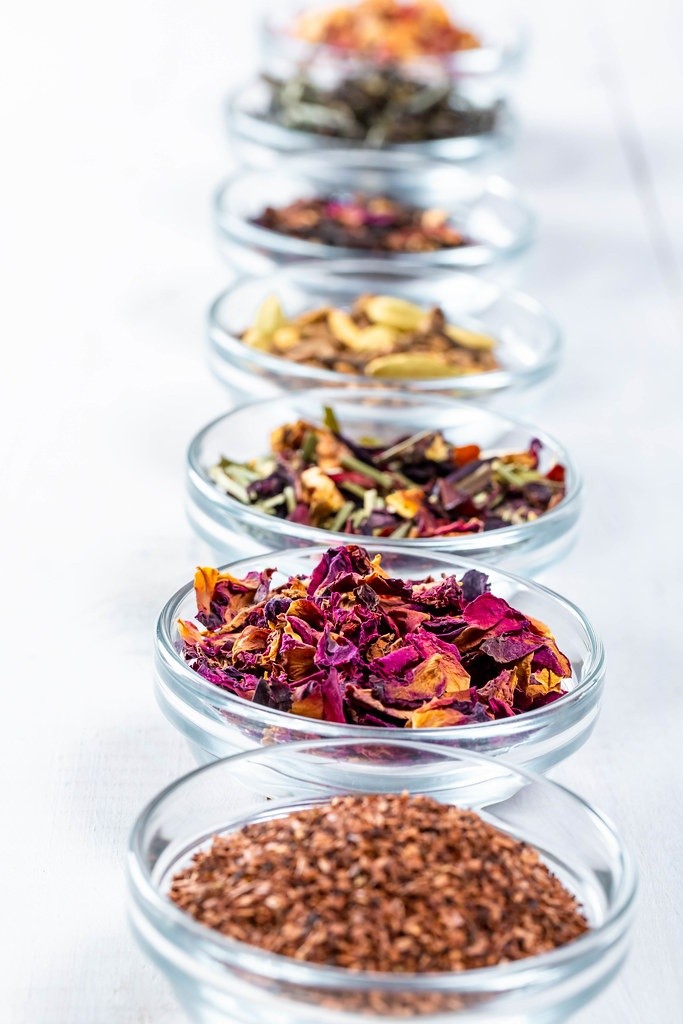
[212,149,532,278]
[153,545,608,809]
[124,739,641,1024]
[205,262,563,404]
[186,389,586,580]
[225,78,522,163]
